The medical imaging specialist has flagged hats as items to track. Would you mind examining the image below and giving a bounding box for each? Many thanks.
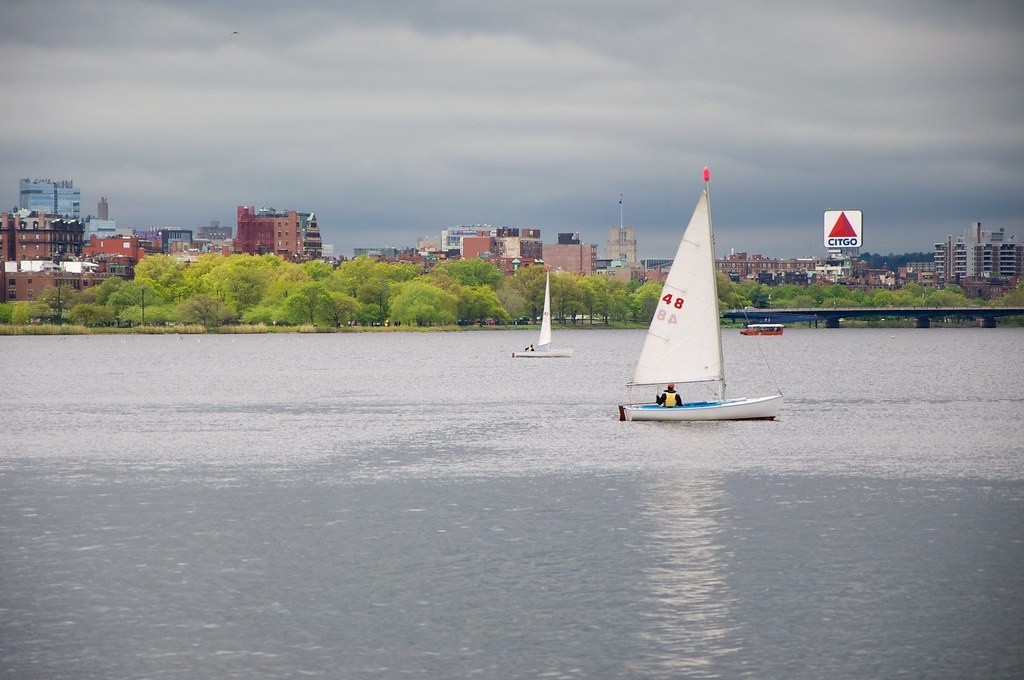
[669,383,674,388]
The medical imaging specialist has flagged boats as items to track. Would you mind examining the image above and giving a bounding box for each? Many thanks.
[740,323,785,336]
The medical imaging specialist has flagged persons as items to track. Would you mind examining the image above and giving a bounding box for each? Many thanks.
[656,383,683,407]
[525,344,534,351]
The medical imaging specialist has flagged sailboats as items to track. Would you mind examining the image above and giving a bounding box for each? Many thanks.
[618,164,787,422]
[512,264,574,357]
